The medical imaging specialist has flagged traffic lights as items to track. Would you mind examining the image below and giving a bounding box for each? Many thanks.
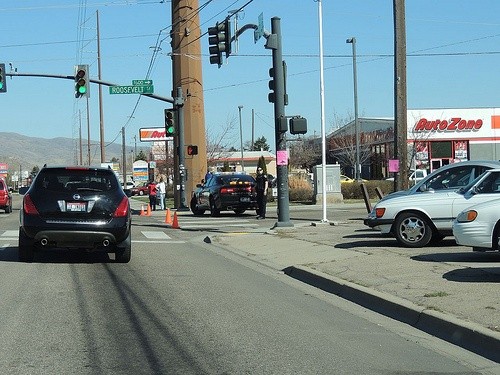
[187,145,199,155]
[165,108,179,137]
[73,64,89,99]
[215,19,231,58]
[207,25,223,69]
[0,63,7,93]
[266,61,288,105]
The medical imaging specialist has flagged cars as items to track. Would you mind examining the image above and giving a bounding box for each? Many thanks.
[451,197,500,254]
[363,161,500,249]
[0,178,12,212]
[190,170,259,219]
[17,166,131,264]
[131,181,158,196]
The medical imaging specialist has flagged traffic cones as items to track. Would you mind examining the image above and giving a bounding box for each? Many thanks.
[139,205,145,215]
[146,203,151,217]
[165,207,172,223]
[171,211,180,229]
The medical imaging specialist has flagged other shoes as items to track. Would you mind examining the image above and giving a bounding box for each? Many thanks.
[256,216,265,219]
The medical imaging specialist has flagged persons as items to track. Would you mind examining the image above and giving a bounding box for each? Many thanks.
[148,178,166,211]
[252,166,269,220]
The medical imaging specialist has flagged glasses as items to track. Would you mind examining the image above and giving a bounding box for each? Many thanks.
[258,170,262,171]
